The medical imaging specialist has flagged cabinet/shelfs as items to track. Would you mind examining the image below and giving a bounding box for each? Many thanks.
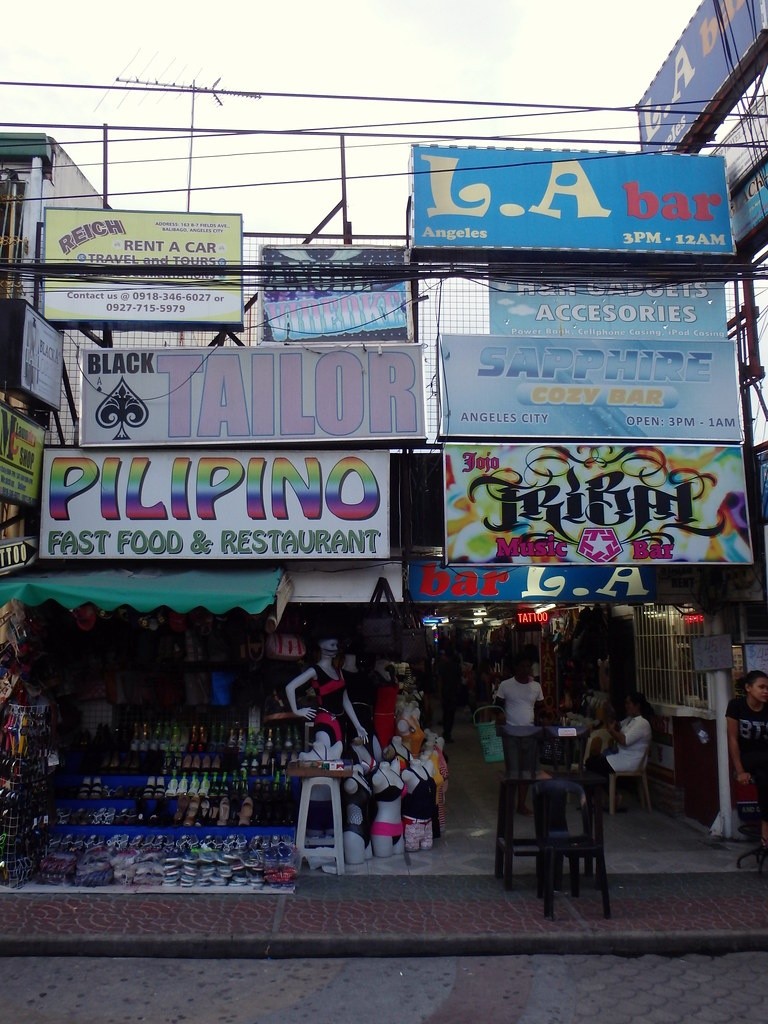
[46,747,301,841]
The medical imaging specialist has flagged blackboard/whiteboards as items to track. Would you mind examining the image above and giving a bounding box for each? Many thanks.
[740,641,768,675]
[691,631,734,673]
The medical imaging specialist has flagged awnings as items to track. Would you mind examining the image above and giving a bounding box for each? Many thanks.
[0,564,290,629]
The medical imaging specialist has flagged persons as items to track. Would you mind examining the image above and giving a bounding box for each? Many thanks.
[285,638,447,862]
[597,692,657,814]
[726,670,768,848]
[491,654,543,815]
[437,647,461,743]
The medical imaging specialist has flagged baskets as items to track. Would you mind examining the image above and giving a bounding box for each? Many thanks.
[473,705,507,763]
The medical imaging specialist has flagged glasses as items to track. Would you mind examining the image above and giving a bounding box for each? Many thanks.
[0,711,46,881]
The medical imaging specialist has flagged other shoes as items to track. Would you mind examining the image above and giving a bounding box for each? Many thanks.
[444,739,454,744]
[517,807,534,816]
[615,790,624,811]
[40,720,297,885]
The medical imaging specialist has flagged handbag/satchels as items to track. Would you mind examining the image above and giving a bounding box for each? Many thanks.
[359,576,428,671]
[265,612,306,660]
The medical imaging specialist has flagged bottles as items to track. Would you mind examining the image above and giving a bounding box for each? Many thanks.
[130,720,301,799]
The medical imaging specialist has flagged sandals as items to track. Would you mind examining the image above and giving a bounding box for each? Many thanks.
[760,836,768,849]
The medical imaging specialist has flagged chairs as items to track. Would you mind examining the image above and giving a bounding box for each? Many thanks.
[495,776,569,891]
[533,778,610,921]
[607,744,653,815]
